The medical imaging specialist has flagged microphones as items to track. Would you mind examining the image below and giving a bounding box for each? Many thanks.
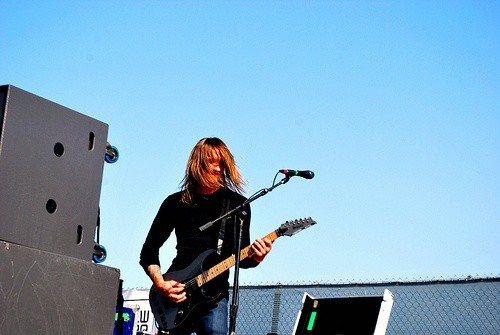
[278,169,315,179]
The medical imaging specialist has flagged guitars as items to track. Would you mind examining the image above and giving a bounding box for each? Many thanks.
[148,217,317,330]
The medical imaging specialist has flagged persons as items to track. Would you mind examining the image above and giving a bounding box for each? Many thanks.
[139,136,273,335]
